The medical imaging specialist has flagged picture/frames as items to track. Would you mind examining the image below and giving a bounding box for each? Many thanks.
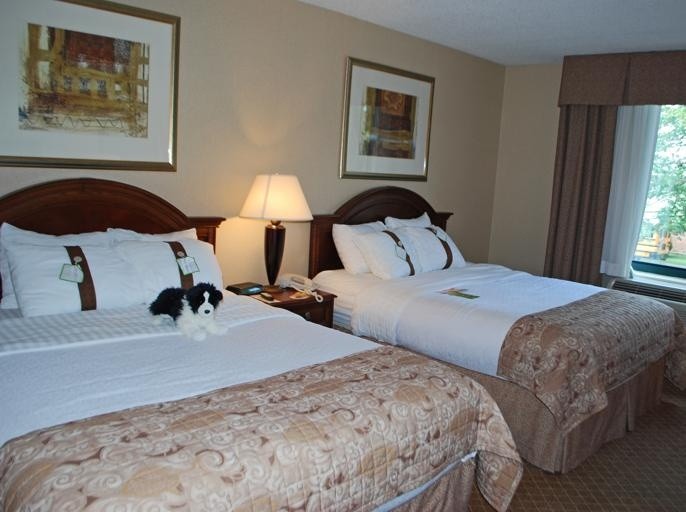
[1,0,181,173]
[338,55,436,182]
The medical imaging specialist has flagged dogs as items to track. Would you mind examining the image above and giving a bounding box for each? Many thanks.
[147,283,229,342]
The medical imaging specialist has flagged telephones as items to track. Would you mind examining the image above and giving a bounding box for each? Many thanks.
[279,272,324,303]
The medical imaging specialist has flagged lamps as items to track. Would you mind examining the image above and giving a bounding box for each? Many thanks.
[238,173,314,294]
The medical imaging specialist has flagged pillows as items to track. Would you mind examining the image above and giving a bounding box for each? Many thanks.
[352,225,466,279]
[109,236,224,305]
[0,238,144,317]
[0,221,112,310]
[107,226,198,241]
[332,220,387,274]
[384,212,432,230]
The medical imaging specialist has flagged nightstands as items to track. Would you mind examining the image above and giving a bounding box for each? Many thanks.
[249,283,338,328]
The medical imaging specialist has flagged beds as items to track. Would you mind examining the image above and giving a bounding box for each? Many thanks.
[307,185,686,475]
[0,176,524,512]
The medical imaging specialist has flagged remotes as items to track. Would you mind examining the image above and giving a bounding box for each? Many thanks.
[260,292,273,300]
